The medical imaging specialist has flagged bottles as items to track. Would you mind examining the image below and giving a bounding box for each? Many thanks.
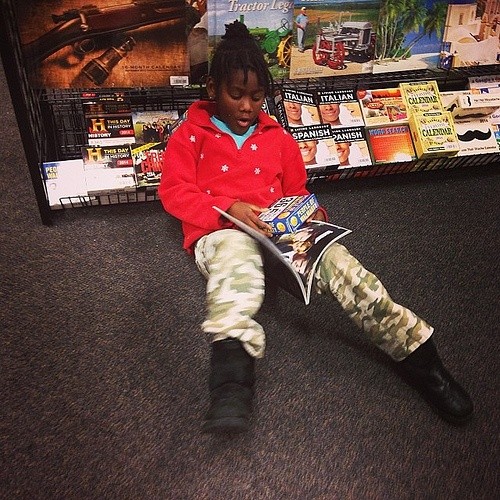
[82,36,136,85]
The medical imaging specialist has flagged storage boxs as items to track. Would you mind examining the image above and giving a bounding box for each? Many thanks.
[0,0,500,224]
[453,118,500,153]
[408,110,458,157]
[445,95,499,116]
[256,194,319,233]
[399,80,443,117]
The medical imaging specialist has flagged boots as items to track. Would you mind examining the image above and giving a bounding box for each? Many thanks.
[201,341,255,433]
[398,337,473,424]
